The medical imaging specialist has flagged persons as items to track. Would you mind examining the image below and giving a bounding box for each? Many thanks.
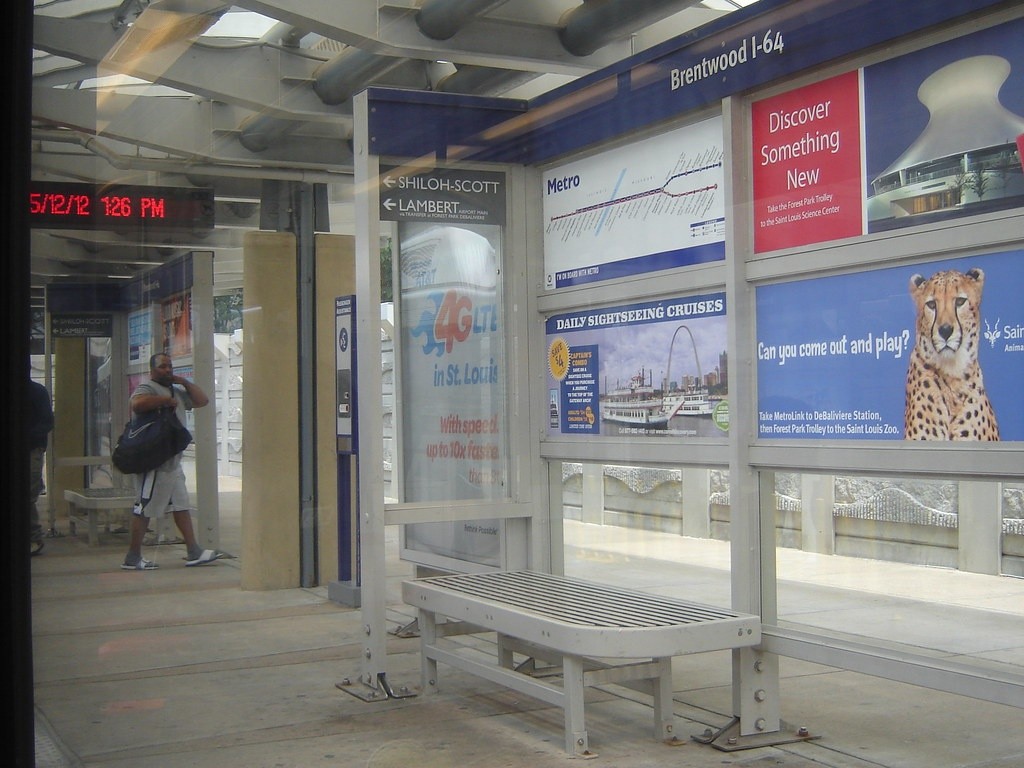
[120,354,223,570]
[30,362,55,553]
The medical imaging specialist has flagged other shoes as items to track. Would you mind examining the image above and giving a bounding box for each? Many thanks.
[30,541,44,553]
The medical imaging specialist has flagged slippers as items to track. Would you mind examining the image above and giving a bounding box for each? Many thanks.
[119,557,160,570]
[184,548,224,567]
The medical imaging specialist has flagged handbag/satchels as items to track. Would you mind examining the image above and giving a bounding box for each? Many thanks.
[111,405,193,475]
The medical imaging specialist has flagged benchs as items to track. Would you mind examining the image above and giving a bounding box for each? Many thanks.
[64,487,140,549]
[402,568,762,764]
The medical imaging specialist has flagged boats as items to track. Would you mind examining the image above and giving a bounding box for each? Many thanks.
[600,366,683,427]
[660,387,714,415]
